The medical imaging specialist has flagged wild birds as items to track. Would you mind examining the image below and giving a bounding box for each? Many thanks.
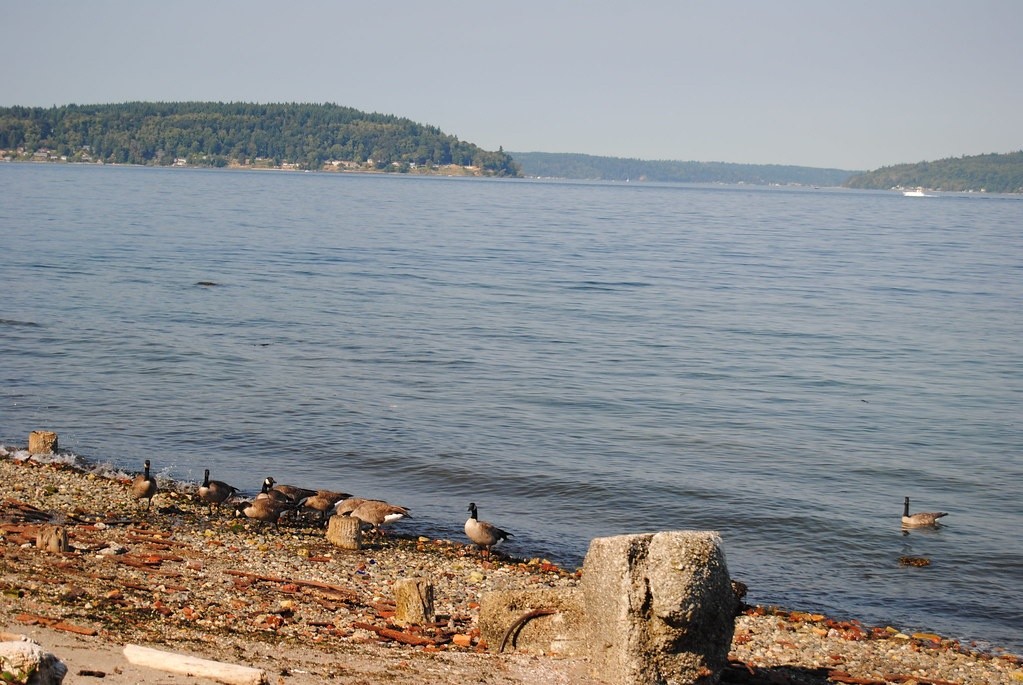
[199,468,241,516]
[131,459,157,507]
[350,501,414,538]
[902,497,949,524]
[465,503,515,562]
[257,475,318,507]
[235,497,300,534]
[301,486,355,511]
[332,498,387,516]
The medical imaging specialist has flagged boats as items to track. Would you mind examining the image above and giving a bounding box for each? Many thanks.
[903,188,925,197]
